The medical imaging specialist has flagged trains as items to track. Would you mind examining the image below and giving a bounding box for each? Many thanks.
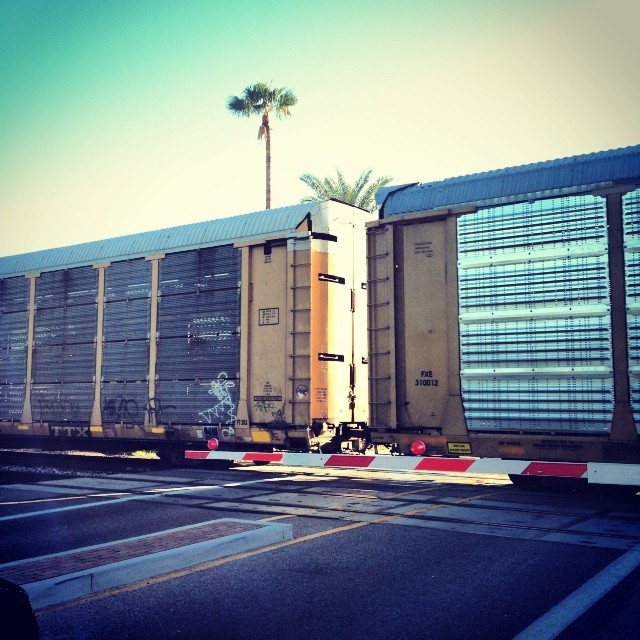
[0,142,640,494]
